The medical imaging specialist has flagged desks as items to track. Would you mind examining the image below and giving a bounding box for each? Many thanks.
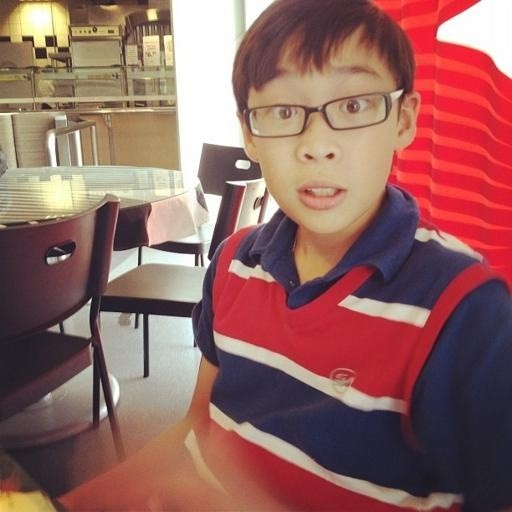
[0,166,212,451]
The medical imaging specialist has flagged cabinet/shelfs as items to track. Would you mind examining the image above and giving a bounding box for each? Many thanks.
[0,66,177,168]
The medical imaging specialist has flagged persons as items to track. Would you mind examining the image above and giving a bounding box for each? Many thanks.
[143,1,511,512]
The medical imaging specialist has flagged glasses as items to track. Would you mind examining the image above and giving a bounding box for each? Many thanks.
[242,86,408,139]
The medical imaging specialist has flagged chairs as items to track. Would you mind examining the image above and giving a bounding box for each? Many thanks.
[134,141,269,327]
[87,176,263,427]
[0,194,125,461]
